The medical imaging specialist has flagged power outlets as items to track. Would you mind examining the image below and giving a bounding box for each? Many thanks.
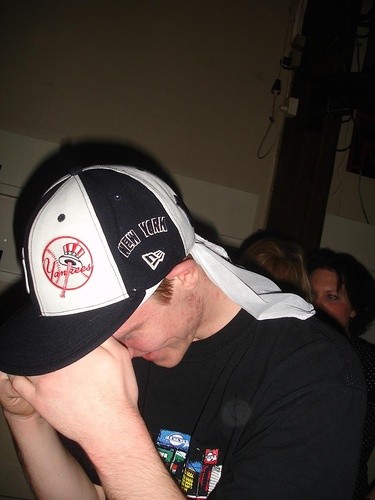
[280,97,299,121]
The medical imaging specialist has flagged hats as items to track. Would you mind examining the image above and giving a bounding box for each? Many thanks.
[1,162,195,377]
